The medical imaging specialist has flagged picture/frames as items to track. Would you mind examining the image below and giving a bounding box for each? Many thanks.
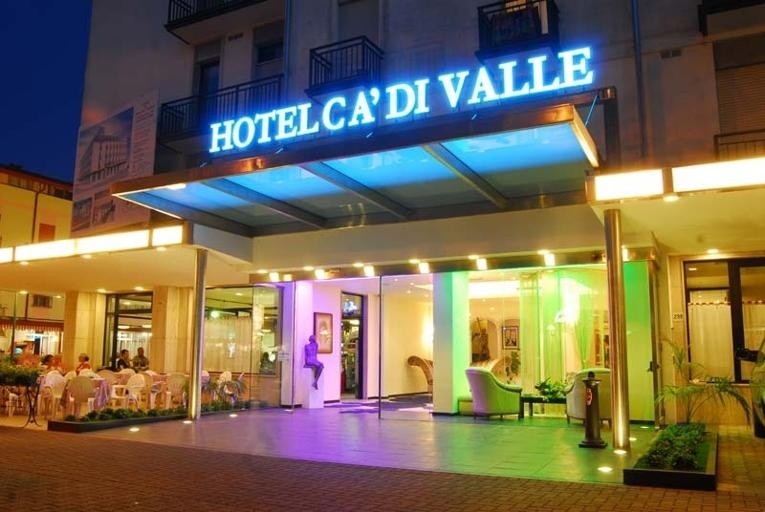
[314,312,333,354]
[502,325,520,350]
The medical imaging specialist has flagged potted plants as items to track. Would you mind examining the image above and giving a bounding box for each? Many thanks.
[0,366,47,428]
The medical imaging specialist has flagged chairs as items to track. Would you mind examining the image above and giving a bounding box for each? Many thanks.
[566,368,611,429]
[465,368,523,421]
[45,369,244,420]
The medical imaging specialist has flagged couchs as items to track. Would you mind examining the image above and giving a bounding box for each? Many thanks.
[407,355,501,393]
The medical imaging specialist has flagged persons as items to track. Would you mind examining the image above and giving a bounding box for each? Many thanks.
[259,352,273,372]
[304,335,324,389]
[75,354,91,375]
[133,347,149,371]
[117,350,131,371]
[42,354,66,376]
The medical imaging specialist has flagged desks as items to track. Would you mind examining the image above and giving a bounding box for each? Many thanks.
[520,394,566,418]
